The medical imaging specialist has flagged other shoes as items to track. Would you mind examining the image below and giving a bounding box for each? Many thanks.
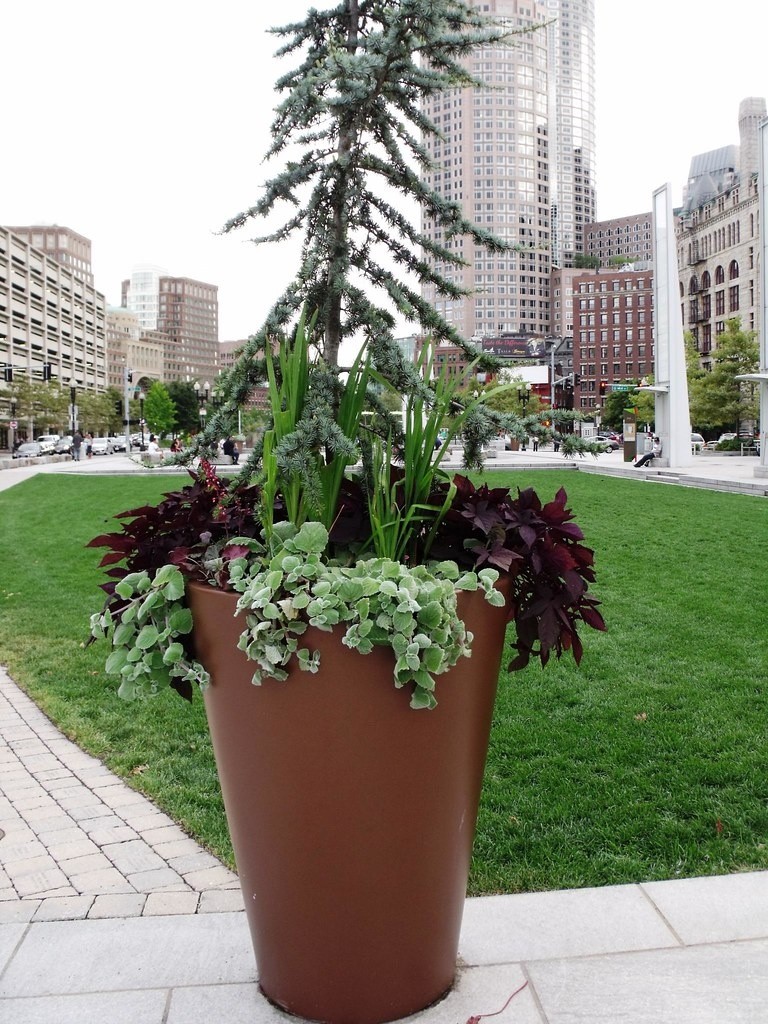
[234,462,239,465]
[645,464,648,467]
[634,465,639,467]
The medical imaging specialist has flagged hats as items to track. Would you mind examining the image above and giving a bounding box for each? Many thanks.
[654,437,659,442]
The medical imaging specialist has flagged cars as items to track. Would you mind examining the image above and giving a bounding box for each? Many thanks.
[717,432,761,445]
[112,432,180,453]
[33,435,91,456]
[91,438,115,456]
[701,440,719,451]
[690,432,706,451]
[583,430,655,454]
[12,442,45,459]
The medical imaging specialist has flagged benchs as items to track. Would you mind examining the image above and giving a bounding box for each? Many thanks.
[644,458,667,467]
[481,450,498,459]
[140,452,160,464]
[199,455,235,464]
[754,466,768,478]
[431,448,452,461]
[165,452,182,459]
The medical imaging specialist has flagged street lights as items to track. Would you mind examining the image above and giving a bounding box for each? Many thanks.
[550,335,573,432]
[516,383,532,451]
[68,377,79,461]
[139,392,146,451]
[193,382,211,432]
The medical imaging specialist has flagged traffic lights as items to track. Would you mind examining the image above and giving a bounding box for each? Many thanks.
[573,372,581,387]
[115,400,122,416]
[599,381,606,395]
[128,372,132,382]
[553,404,559,410]
[544,418,551,427]
[563,379,570,392]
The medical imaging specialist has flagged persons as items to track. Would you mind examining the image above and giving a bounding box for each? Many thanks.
[553,432,563,451]
[700,442,707,453]
[149,433,164,465]
[610,433,623,443]
[441,429,446,440]
[13,436,29,453]
[533,436,538,452]
[434,437,442,450]
[212,436,239,466]
[71,430,93,462]
[634,437,661,467]
[171,438,184,454]
[649,431,656,451]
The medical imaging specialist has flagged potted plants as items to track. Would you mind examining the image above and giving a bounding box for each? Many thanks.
[85,313,607,1024]
